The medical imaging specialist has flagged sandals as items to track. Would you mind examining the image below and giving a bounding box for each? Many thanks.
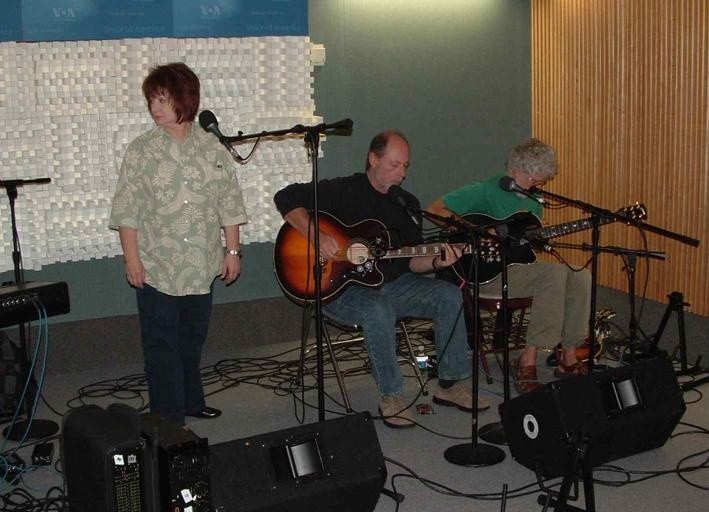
[554,360,589,378]
[509,358,541,395]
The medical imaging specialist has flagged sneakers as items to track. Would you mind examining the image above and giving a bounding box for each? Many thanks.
[378,394,415,429]
[432,380,490,412]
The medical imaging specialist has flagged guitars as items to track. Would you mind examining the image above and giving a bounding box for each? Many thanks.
[441,201,647,289]
[273,209,471,306]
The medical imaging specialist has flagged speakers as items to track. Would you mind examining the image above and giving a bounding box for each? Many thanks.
[139,414,209,512]
[61,403,147,512]
[498,350,687,479]
[207,409,388,512]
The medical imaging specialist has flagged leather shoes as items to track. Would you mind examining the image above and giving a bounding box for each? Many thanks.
[192,406,221,418]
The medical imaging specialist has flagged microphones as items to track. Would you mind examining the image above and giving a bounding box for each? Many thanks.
[498,175,546,205]
[533,236,552,252]
[199,110,243,164]
[387,184,422,231]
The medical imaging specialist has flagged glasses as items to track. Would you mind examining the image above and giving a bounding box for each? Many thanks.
[529,178,546,188]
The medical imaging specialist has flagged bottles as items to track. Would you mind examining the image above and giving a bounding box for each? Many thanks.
[414,343,430,388]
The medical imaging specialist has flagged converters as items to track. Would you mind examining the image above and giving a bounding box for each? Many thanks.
[1,453,25,474]
[31,442,55,466]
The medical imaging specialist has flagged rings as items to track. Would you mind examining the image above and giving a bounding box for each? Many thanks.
[445,256,449,260]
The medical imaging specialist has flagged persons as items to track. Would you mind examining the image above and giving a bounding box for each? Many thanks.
[110,62,249,429]
[273,131,491,428]
[428,138,594,394]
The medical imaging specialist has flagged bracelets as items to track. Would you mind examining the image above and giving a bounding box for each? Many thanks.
[225,248,242,257]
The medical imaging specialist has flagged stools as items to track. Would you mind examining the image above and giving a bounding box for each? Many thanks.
[307,309,430,414]
[463,284,561,386]
